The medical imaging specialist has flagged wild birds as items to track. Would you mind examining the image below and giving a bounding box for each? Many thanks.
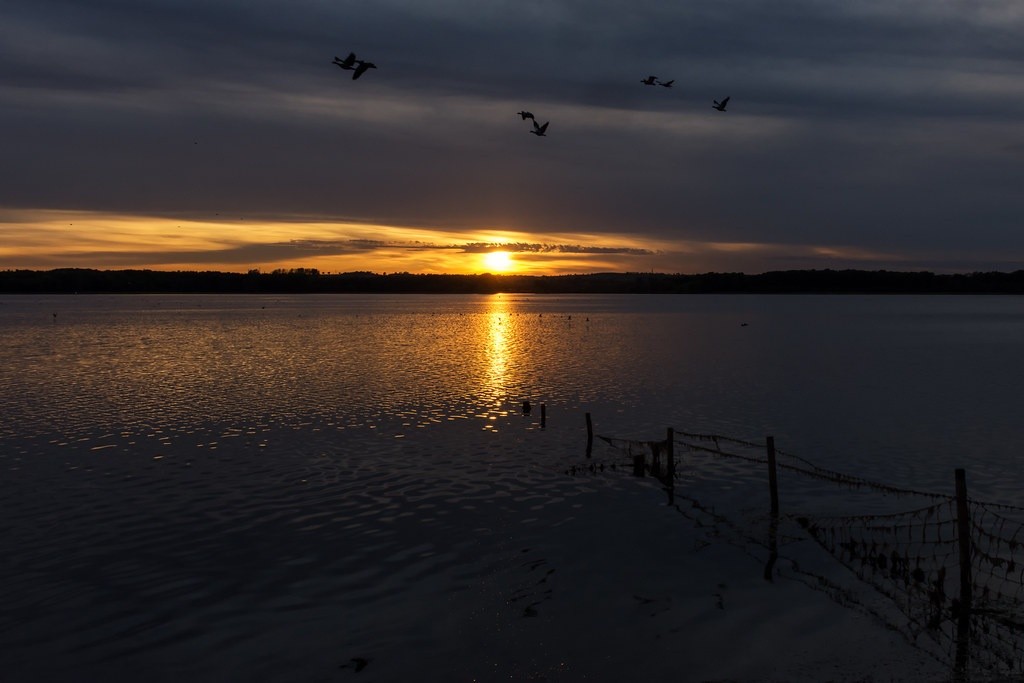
[331,52,377,80]
[712,97,730,112]
[517,111,550,136]
[641,76,675,88]
